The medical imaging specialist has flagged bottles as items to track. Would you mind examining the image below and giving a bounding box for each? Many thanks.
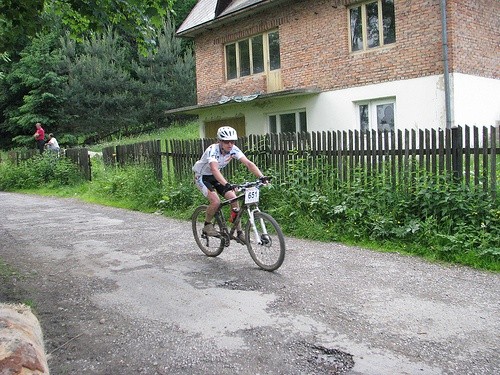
[230,207,239,223]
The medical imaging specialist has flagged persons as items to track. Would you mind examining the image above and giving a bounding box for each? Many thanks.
[45,133,60,153]
[193,126,268,244]
[34,123,45,155]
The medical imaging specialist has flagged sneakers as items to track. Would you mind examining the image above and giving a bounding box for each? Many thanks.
[202,224,221,236]
[237,234,254,243]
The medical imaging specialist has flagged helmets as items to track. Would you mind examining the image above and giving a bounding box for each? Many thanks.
[217,126,238,141]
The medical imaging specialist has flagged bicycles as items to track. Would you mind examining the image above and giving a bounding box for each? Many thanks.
[192,176,286,272]
